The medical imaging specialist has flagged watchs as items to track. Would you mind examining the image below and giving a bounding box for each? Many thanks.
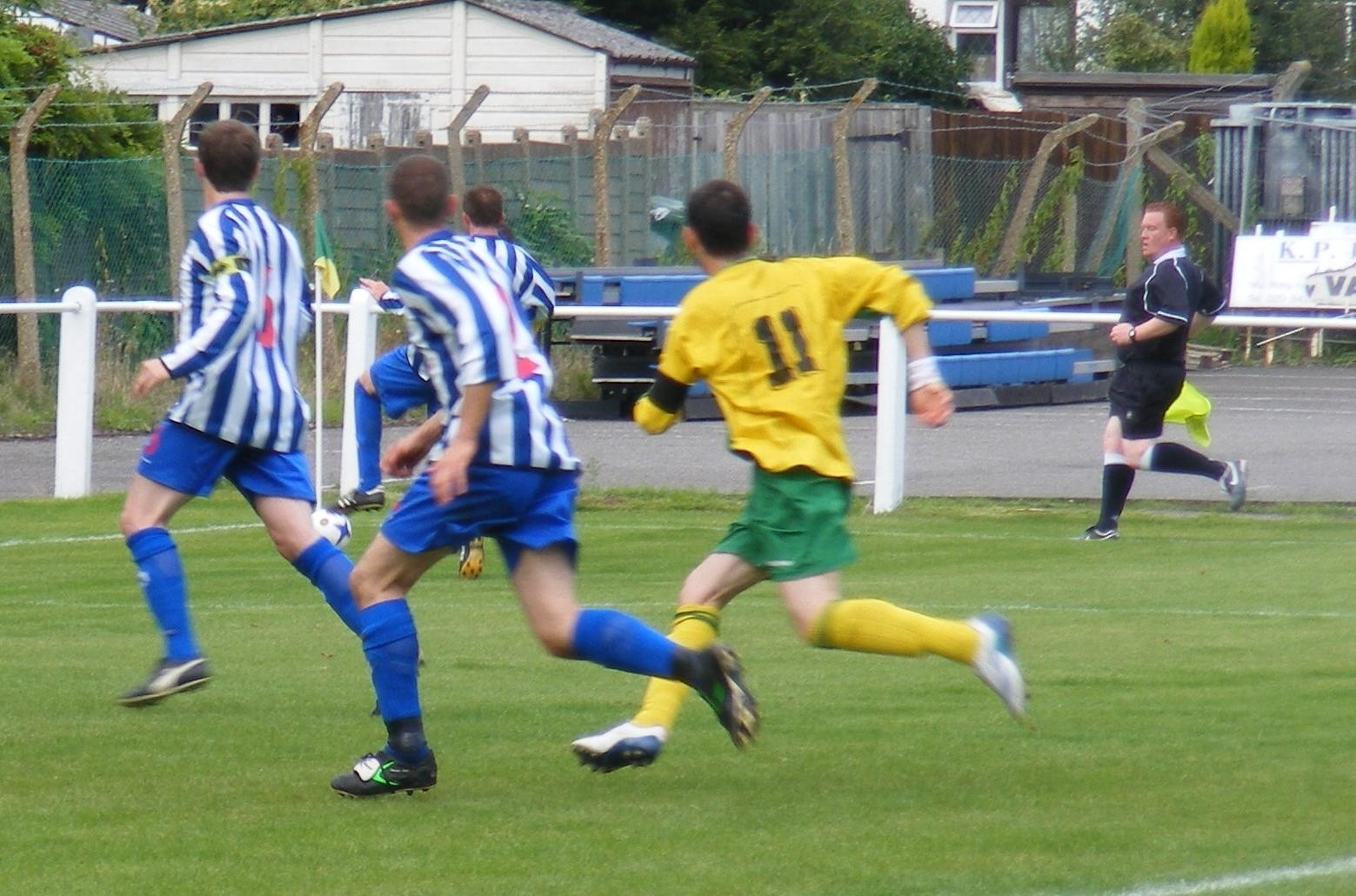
[1128,326,1136,343]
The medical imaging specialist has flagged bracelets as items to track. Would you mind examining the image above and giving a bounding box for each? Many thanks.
[906,356,942,393]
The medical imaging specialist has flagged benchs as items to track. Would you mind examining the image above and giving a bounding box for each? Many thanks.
[546,268,1076,397]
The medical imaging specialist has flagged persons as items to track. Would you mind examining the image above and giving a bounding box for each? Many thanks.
[1070,198,1250,543]
[115,119,423,720]
[328,182,556,582]
[497,224,513,242]
[331,154,760,798]
[567,180,1024,773]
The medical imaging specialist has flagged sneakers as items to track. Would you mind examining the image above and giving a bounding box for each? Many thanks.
[115,657,214,708]
[966,611,1030,725]
[329,750,438,800]
[331,484,385,515]
[692,645,760,748]
[459,536,483,581]
[1082,524,1121,542]
[570,721,667,774]
[1218,459,1248,510]
